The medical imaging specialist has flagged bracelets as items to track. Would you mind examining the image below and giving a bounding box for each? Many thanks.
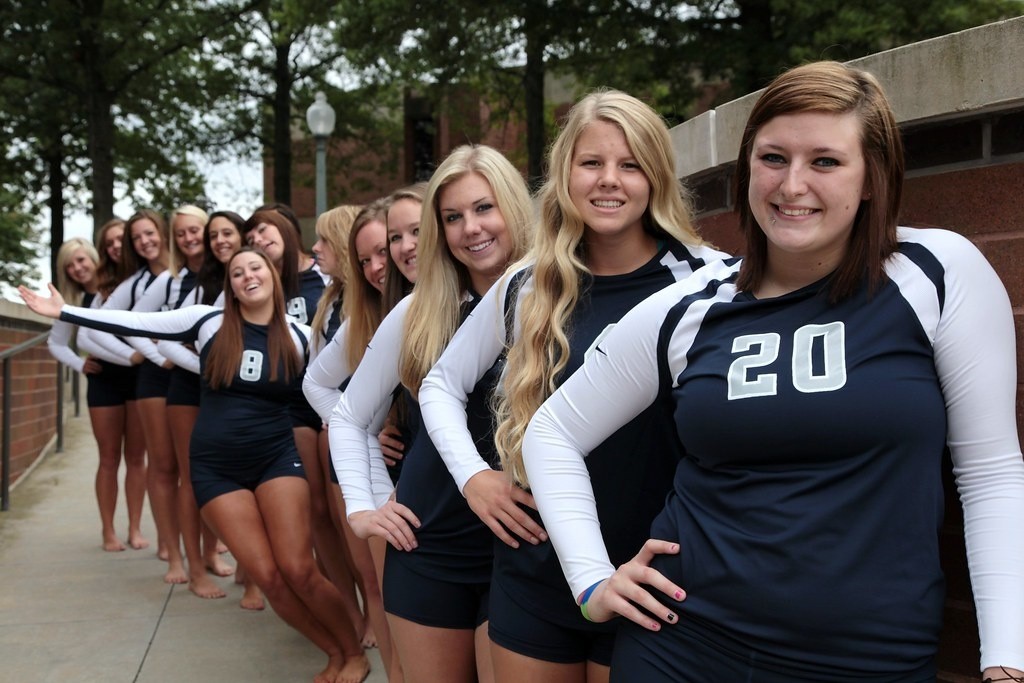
[578,580,604,621]
[981,665,1024,683]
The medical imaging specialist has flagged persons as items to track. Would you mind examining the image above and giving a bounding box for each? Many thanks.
[418,89,733,683]
[328,146,535,683]
[522,60,1024,683]
[19,248,371,683]
[46,184,427,649]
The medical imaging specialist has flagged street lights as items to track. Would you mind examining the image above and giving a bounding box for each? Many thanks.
[305,90,335,238]
